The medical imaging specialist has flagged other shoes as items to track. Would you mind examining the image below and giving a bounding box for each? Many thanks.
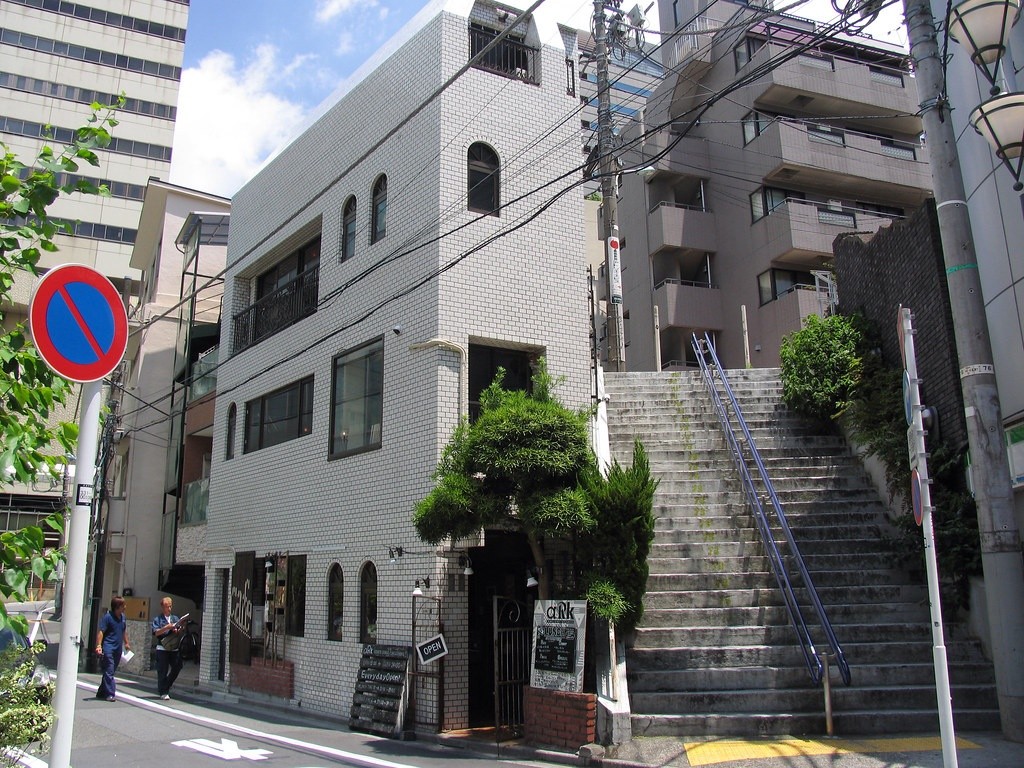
[103,695,116,701]
[96,694,104,699]
[161,694,170,700]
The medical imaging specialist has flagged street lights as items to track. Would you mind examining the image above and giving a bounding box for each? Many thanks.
[603,167,656,373]
[930,0,1024,737]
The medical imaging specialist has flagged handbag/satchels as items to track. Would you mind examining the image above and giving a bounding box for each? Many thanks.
[95,654,105,667]
[161,633,179,650]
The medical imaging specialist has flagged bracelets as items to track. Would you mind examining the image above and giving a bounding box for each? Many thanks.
[96,645,102,647]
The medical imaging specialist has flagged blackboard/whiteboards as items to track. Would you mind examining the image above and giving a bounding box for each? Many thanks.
[348,644,412,736]
[534,625,578,673]
[415,633,448,665]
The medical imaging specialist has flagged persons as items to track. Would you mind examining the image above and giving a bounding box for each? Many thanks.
[151,596,184,701]
[94,595,130,702]
